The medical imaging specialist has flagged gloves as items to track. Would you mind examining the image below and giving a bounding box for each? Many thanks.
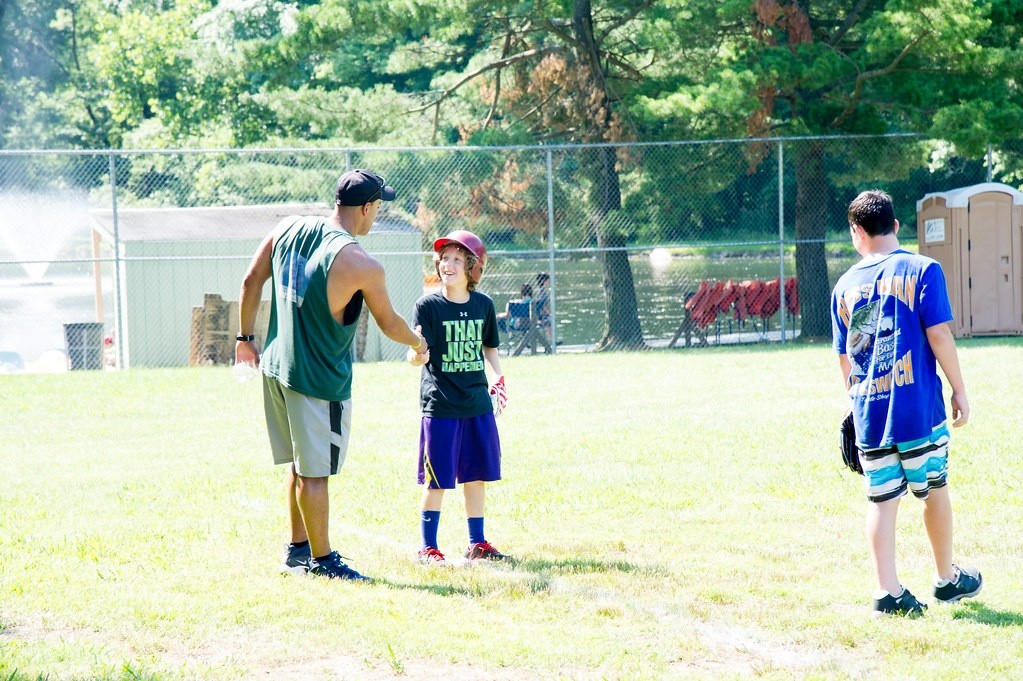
[489,377,507,417]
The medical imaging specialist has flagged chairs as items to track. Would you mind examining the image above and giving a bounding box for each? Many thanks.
[499,300,552,356]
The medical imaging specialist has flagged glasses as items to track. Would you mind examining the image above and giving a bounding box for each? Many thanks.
[365,175,385,209]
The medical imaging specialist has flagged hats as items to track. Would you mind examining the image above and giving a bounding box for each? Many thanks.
[336,169,396,206]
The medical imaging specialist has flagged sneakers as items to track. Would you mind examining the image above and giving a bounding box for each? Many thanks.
[875,585,928,616]
[418,546,456,574]
[935,563,983,603]
[308,551,371,584]
[466,540,511,563]
[286,542,353,568]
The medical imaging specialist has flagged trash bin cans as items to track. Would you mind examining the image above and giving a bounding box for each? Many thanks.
[64,322,103,373]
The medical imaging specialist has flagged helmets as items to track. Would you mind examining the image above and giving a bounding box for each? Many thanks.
[840,413,865,476]
[433,230,487,270]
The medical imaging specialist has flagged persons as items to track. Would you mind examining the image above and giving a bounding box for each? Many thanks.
[235,168,428,578]
[496,274,563,344]
[407,230,507,567]
[830,188,983,616]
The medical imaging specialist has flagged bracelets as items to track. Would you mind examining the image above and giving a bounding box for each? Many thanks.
[235,333,254,341]
[411,335,422,349]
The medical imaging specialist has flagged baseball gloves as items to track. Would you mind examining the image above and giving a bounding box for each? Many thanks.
[487,376,509,417]
[840,408,866,476]
